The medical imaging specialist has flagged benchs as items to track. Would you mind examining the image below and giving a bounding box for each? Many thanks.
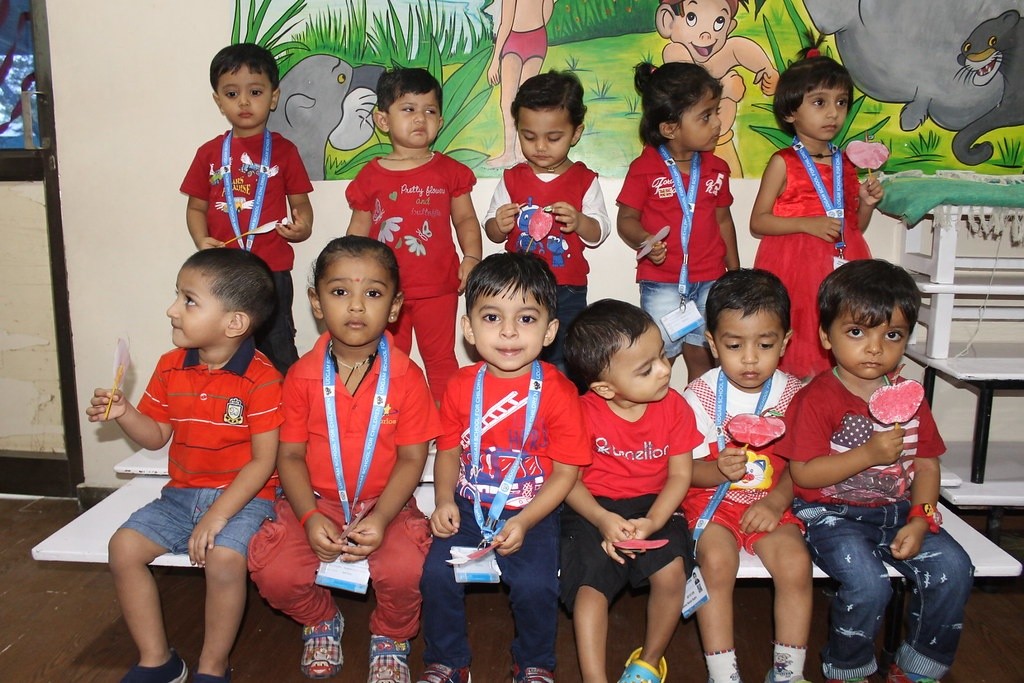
[31,437,1021,678]
[878,175,1023,592]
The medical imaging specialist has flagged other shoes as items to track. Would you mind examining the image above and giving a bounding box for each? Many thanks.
[414,657,474,683]
[119,647,189,683]
[510,662,555,683]
[190,659,231,683]
[885,663,941,683]
[366,633,411,683]
[300,607,344,678]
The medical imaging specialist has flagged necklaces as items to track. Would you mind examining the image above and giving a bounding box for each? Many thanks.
[383,156,432,160]
[329,340,378,395]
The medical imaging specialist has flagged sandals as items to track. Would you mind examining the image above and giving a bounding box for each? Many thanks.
[615,647,667,683]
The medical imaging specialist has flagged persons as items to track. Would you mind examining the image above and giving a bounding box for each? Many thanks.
[560,300,705,683]
[772,259,975,683]
[344,68,483,406]
[179,43,313,375]
[749,27,883,380]
[418,251,592,683]
[86,248,285,683]
[482,70,611,372]
[682,268,813,683]
[616,61,740,383]
[246,233,444,683]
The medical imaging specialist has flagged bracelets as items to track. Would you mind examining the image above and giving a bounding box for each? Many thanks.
[463,256,481,262]
[300,509,319,527]
[906,503,943,534]
